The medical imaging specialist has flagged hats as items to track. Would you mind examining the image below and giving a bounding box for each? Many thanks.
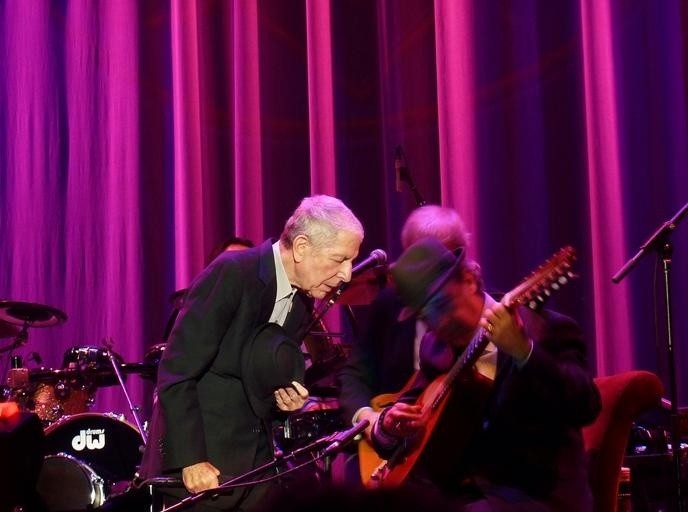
[385,234,467,323]
[239,321,306,422]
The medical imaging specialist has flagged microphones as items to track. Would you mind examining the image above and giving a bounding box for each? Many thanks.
[352,249,387,276]
[64,347,109,360]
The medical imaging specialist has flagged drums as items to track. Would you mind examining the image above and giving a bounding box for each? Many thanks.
[61,345,126,387]
[15,452,106,512]
[0,368,94,427]
[141,342,167,383]
[43,412,147,500]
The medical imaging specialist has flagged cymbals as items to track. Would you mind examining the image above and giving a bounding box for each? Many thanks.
[0,300,67,328]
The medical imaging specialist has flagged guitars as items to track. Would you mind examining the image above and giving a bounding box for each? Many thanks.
[358,245,581,490]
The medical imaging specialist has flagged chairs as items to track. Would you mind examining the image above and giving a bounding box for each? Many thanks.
[581,370,665,512]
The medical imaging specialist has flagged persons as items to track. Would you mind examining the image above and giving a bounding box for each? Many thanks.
[139,194,603,511]
